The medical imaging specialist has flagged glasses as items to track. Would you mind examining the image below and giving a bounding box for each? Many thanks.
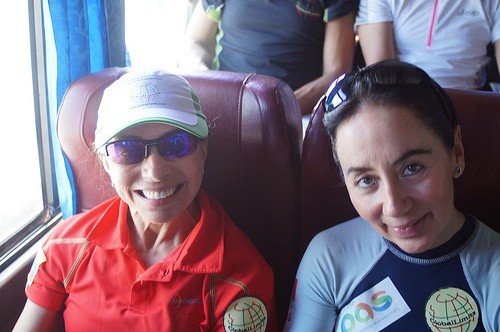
[323,65,456,122]
[105,129,201,165]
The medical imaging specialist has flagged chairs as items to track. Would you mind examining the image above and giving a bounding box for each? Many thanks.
[57,66,500,332]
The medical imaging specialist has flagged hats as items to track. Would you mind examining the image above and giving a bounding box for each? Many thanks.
[93,68,208,153]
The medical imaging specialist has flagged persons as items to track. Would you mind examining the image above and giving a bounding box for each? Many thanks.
[284,58,500,332]
[11,67,273,332]
[184,0,359,114]
[353,0,500,91]
